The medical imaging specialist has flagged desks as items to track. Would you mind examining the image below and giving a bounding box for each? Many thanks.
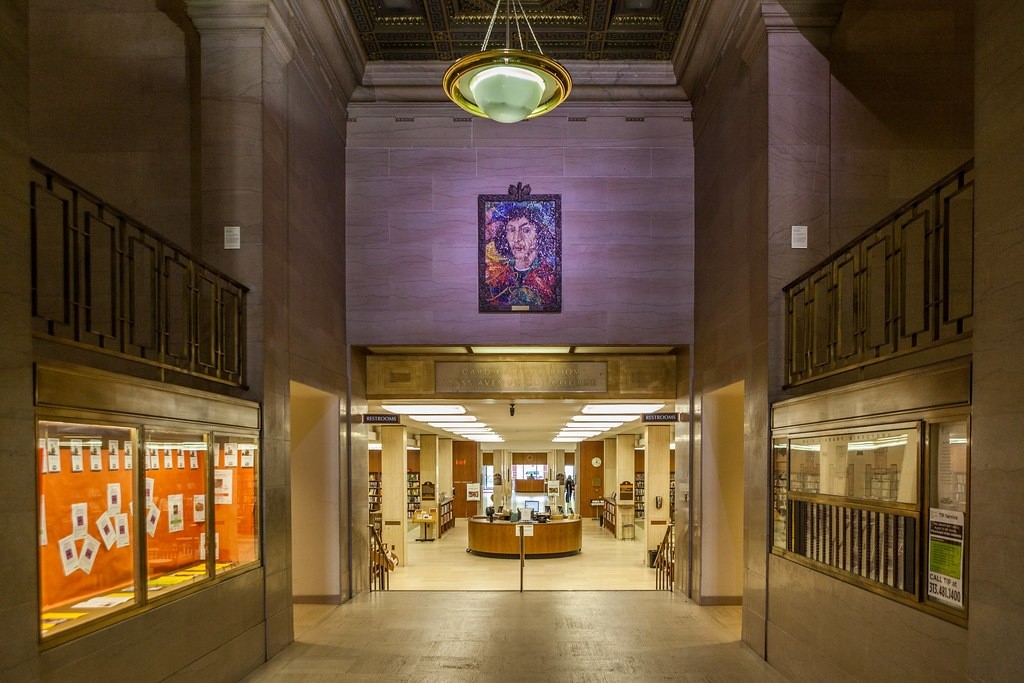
[413,519,435,542]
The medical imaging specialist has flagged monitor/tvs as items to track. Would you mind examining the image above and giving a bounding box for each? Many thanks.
[517,501,539,523]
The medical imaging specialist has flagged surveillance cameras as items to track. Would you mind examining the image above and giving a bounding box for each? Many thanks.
[510,412,514,416]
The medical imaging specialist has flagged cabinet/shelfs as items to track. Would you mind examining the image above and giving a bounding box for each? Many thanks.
[604,497,617,538]
[670,471,676,523]
[439,498,455,538]
[776,470,820,515]
[368,471,382,535]
[407,472,420,519]
[635,472,645,519]
[34,414,262,650]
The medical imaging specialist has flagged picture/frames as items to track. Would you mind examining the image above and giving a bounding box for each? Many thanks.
[477,194,564,314]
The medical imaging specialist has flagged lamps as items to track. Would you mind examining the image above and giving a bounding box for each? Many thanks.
[442,0,573,124]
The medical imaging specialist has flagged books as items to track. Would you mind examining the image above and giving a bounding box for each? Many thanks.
[635,474,644,517]
[670,473,676,509]
[441,502,453,525]
[369,475,382,510]
[955,474,966,502]
[408,474,421,518]
[603,500,616,525]
[774,471,821,509]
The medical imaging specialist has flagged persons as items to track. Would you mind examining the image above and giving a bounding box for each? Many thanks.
[565,475,574,500]
[529,474,534,479]
